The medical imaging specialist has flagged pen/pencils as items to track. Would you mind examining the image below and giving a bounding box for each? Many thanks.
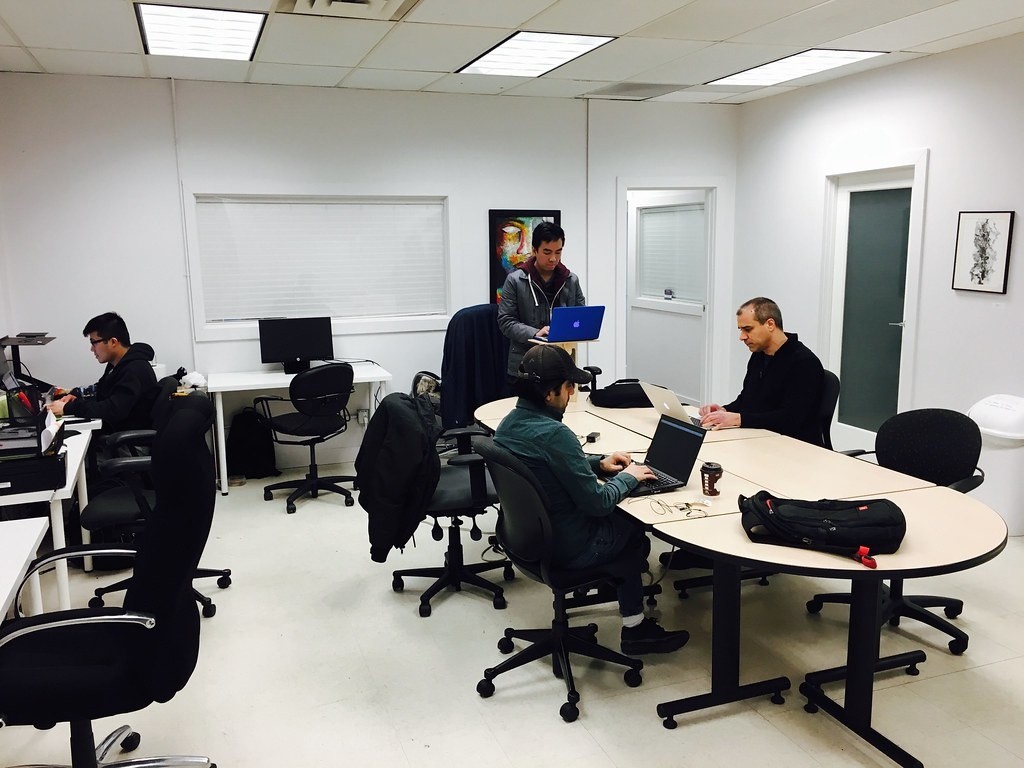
[62,442,67,447]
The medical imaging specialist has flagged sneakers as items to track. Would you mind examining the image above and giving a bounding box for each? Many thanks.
[620,617,690,656]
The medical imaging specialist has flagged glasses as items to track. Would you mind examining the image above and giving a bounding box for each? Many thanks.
[90,339,104,345]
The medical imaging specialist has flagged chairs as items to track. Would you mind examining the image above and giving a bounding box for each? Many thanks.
[806,408,985,656]
[0,303,843,768]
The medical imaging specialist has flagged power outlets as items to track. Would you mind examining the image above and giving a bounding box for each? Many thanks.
[357,409,368,425]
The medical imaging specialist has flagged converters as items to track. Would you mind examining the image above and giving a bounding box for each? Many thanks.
[587,432,601,441]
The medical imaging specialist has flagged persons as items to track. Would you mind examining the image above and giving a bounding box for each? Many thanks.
[498,222,584,395]
[495,344,689,655]
[659,297,824,570]
[43,311,159,566]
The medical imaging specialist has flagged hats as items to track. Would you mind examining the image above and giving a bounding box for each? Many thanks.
[519,344,593,384]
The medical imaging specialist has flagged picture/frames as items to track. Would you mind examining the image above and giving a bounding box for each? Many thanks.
[952,211,1016,295]
[488,208,560,302]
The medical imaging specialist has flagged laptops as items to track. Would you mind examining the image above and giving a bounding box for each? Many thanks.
[2,370,20,390]
[640,381,740,430]
[595,413,706,496]
[534,306,605,342]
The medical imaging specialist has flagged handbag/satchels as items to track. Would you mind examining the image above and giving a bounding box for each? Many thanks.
[739,490,906,569]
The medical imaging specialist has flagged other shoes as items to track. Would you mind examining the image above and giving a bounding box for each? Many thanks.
[660,549,714,571]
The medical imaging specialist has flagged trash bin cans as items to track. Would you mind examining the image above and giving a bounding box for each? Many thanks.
[964,394,1024,537]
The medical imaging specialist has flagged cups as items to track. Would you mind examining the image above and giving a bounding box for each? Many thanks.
[700,462,723,496]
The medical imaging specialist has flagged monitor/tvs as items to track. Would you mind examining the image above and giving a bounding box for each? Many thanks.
[259,317,334,374]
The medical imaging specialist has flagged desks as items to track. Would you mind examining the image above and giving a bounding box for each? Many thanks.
[476,391,1009,768]
[0,516,49,633]
[0,430,94,613]
[65,417,102,572]
[209,361,395,497]
